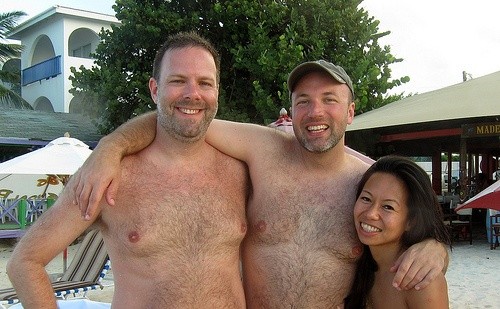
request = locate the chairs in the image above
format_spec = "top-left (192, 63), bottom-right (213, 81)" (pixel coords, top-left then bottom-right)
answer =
top-left (441, 191), bottom-right (500, 250)
top-left (0, 230), bottom-right (111, 309)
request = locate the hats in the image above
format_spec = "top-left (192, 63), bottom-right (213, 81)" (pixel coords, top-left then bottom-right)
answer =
top-left (287, 60), bottom-right (354, 102)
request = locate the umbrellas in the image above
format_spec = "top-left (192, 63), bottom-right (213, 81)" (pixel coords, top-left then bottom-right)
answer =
top-left (0, 132), bottom-right (93, 273)
top-left (455, 179), bottom-right (500, 212)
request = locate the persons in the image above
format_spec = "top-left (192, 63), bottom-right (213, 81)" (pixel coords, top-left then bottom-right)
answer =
top-left (7, 30), bottom-right (253, 309)
top-left (343, 155), bottom-right (449, 309)
top-left (70, 60), bottom-right (449, 309)
